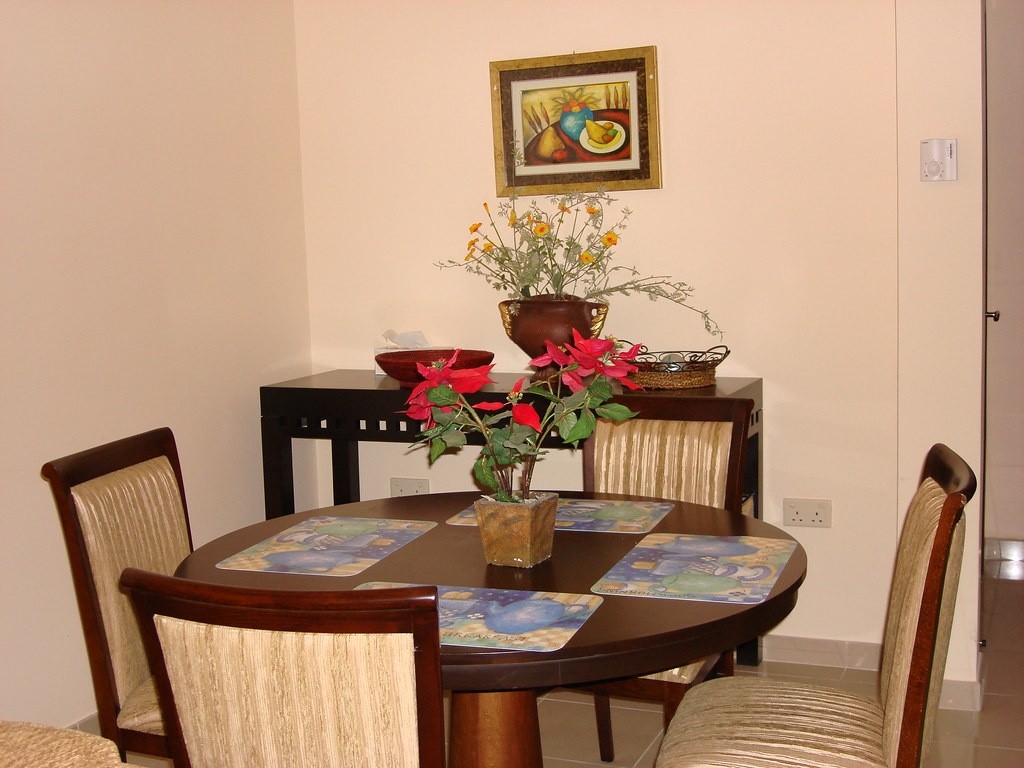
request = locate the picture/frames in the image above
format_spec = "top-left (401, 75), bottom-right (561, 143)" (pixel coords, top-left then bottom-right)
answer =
top-left (488, 46), bottom-right (662, 198)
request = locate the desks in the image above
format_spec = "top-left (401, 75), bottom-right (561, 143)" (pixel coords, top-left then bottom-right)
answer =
top-left (174, 491), bottom-right (808, 768)
top-left (261, 369), bottom-right (770, 526)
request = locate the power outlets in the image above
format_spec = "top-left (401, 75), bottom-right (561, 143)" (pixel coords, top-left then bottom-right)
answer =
top-left (783, 497), bottom-right (833, 528)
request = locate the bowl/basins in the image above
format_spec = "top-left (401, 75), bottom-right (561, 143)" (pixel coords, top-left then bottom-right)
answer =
top-left (375, 348), bottom-right (495, 389)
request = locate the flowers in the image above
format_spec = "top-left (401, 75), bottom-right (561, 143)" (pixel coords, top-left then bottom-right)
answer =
top-left (432, 130), bottom-right (734, 342)
top-left (392, 327), bottom-right (643, 500)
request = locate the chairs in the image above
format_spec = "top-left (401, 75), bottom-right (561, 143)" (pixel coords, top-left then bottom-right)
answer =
top-left (655, 443), bottom-right (977, 768)
top-left (119, 564), bottom-right (444, 768)
top-left (41, 427), bottom-right (222, 767)
top-left (581, 385), bottom-right (754, 761)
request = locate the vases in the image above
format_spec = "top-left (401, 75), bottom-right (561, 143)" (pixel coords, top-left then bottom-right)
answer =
top-left (498, 292), bottom-right (608, 382)
top-left (471, 489), bottom-right (560, 567)
